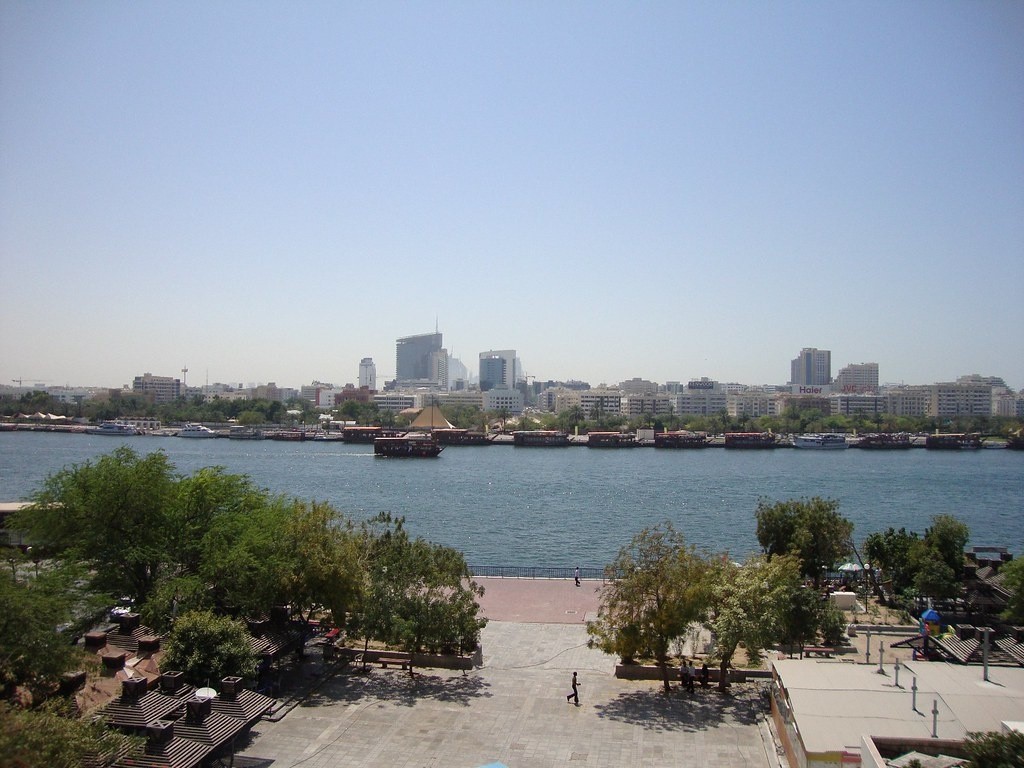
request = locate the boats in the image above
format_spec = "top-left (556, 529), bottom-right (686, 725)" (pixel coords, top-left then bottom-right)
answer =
top-left (430, 428), bottom-right (492, 446)
top-left (655, 431), bottom-right (711, 449)
top-left (724, 432), bottom-right (777, 449)
top-left (512, 430), bottom-right (571, 447)
top-left (151, 422), bottom-right (259, 440)
top-left (790, 432), bottom-right (849, 449)
top-left (586, 429), bottom-right (645, 448)
top-left (373, 437), bottom-right (442, 458)
top-left (84, 420), bottom-right (143, 437)
top-left (344, 425), bottom-right (397, 444)
top-left (926, 431), bottom-right (988, 448)
top-left (858, 429), bottom-right (914, 449)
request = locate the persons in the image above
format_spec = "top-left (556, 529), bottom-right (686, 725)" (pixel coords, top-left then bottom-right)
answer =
top-left (687, 661), bottom-right (695, 694)
top-left (567, 672), bottom-right (581, 707)
top-left (574, 567), bottom-right (581, 587)
top-left (681, 661), bottom-right (688, 688)
top-left (701, 664), bottom-right (710, 691)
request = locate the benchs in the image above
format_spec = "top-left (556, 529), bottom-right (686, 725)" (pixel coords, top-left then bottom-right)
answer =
top-left (676, 675), bottom-right (712, 683)
top-left (377, 658), bottom-right (410, 670)
top-left (308, 621), bottom-right (340, 643)
top-left (803, 647), bottom-right (834, 658)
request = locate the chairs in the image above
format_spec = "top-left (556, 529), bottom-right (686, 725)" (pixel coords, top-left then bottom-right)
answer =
top-left (353, 652), bottom-right (364, 666)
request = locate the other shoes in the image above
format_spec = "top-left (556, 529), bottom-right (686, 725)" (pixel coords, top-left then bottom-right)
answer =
top-left (575, 584), bottom-right (578, 585)
top-left (567, 696), bottom-right (569, 701)
top-left (579, 582), bottom-right (580, 584)
